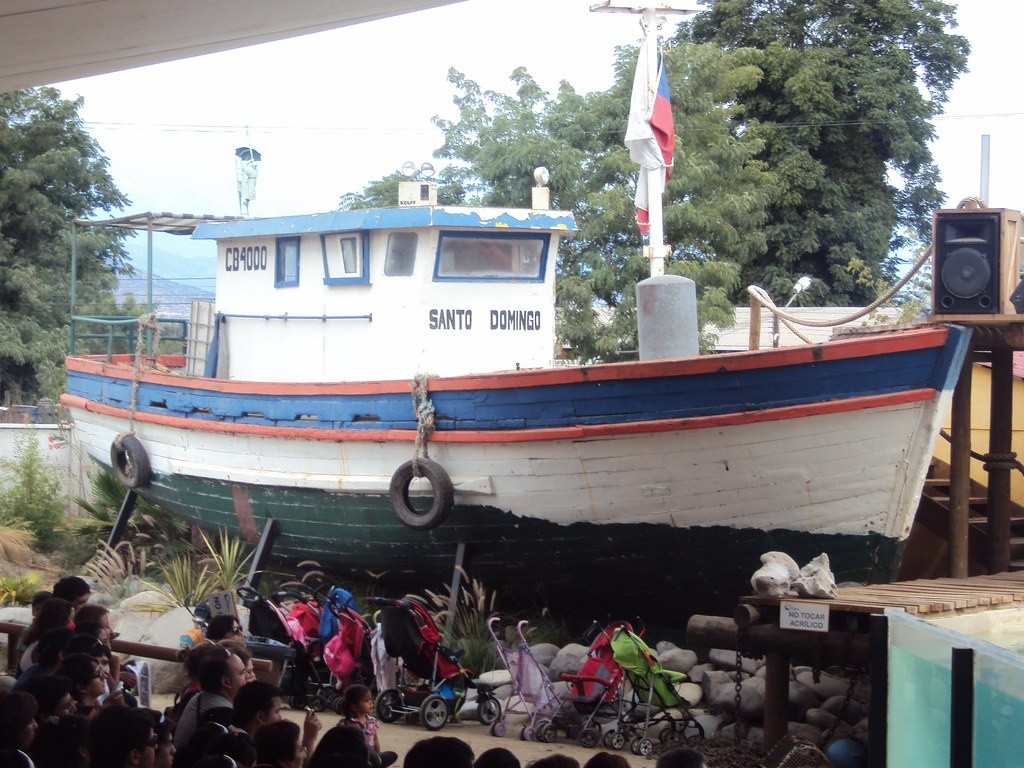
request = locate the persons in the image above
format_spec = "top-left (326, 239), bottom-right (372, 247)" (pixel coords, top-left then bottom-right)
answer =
top-left (336, 684), bottom-right (398, 768)
top-left (656, 747), bottom-right (707, 768)
top-left (474, 747), bottom-right (521, 768)
top-left (403, 736), bottom-right (475, 768)
top-left (307, 726), bottom-right (368, 768)
top-left (525, 753), bottom-right (581, 768)
top-left (583, 751), bottom-right (631, 768)
top-left (0, 576), bottom-right (322, 768)
top-left (253, 720), bottom-right (307, 768)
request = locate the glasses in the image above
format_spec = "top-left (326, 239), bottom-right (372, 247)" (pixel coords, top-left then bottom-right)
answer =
top-left (222, 755), bottom-right (237, 768)
top-left (223, 647), bottom-right (231, 657)
top-left (205, 638), bottom-right (217, 646)
top-left (93, 638), bottom-right (103, 648)
top-left (89, 665), bottom-right (103, 678)
top-left (206, 720), bottom-right (229, 737)
top-left (229, 626), bottom-right (242, 633)
top-left (145, 734), bottom-right (157, 747)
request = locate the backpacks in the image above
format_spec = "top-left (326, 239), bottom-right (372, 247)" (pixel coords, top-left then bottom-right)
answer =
top-left (287, 617), bottom-right (310, 649)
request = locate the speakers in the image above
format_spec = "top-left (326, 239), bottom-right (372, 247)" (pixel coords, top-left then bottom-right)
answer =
top-left (930, 208), bottom-right (1021, 323)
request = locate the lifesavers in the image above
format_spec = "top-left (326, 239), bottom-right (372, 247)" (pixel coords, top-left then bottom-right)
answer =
top-left (109, 433), bottom-right (152, 490)
top-left (389, 457), bottom-right (456, 533)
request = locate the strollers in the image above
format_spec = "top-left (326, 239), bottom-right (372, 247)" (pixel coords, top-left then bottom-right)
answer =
top-left (176, 562), bottom-right (707, 758)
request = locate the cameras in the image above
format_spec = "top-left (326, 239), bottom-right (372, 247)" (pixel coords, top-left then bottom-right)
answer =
top-left (306, 708), bottom-right (314, 717)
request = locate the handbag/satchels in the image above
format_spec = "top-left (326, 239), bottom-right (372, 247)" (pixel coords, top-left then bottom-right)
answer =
top-left (323, 636), bottom-right (356, 679)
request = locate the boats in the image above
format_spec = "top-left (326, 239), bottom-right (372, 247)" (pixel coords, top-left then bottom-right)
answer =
top-left (59, 205), bottom-right (975, 647)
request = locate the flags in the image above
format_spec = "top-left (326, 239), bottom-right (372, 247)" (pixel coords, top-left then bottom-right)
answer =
top-left (622, 37), bottom-right (675, 237)
